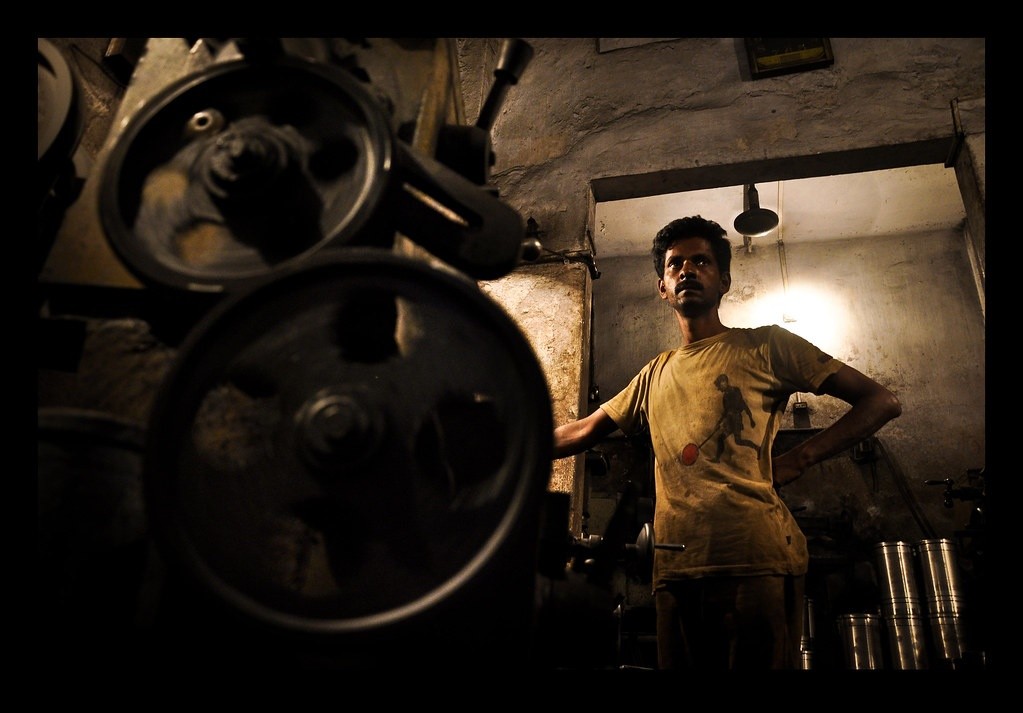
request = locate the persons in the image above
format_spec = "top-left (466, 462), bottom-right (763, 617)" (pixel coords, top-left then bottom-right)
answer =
top-left (554, 216), bottom-right (904, 713)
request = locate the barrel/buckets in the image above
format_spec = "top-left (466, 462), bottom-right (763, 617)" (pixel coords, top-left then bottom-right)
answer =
top-left (839, 537), bottom-right (968, 671)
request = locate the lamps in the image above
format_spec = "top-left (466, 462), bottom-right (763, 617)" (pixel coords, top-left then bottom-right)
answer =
top-left (733, 182), bottom-right (780, 238)
